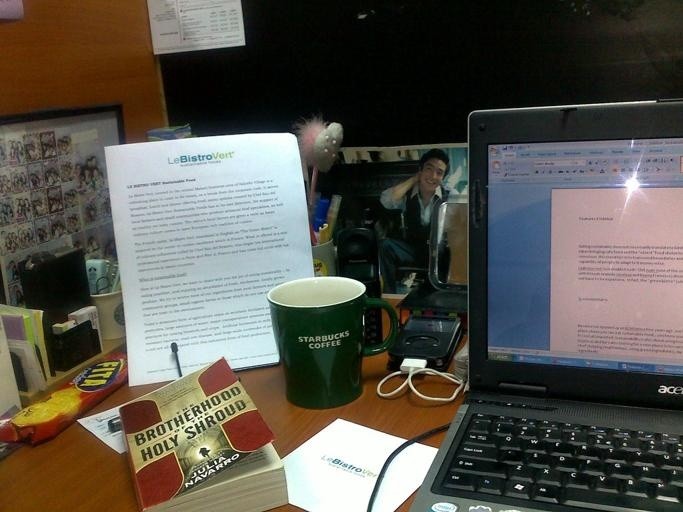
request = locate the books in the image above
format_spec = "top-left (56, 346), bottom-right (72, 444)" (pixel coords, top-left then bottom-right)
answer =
top-left (119, 357), bottom-right (290, 512)
top-left (0, 304), bottom-right (54, 414)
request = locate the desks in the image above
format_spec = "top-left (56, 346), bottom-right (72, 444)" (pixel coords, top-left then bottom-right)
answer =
top-left (0, 296), bottom-right (472, 512)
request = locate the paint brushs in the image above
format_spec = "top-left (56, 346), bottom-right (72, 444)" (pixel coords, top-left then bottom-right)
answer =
top-left (328, 195), bottom-right (342, 238)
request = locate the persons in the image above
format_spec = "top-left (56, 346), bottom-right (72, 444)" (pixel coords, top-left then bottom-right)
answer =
top-left (374, 148), bottom-right (463, 296)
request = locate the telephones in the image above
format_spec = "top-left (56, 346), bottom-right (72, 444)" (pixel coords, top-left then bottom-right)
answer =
top-left (338, 216), bottom-right (383, 347)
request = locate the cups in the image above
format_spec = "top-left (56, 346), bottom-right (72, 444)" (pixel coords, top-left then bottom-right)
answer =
top-left (267, 277), bottom-right (398, 409)
top-left (90, 290), bottom-right (125, 340)
top-left (311, 238), bottom-right (337, 277)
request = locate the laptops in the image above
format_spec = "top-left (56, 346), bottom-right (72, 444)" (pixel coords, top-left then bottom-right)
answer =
top-left (411, 97), bottom-right (683, 511)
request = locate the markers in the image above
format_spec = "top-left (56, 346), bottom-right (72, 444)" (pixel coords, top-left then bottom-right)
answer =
top-left (313, 200), bottom-right (329, 242)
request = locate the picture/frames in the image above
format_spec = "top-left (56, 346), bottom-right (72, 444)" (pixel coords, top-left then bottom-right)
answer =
top-left (1, 103), bottom-right (128, 304)
